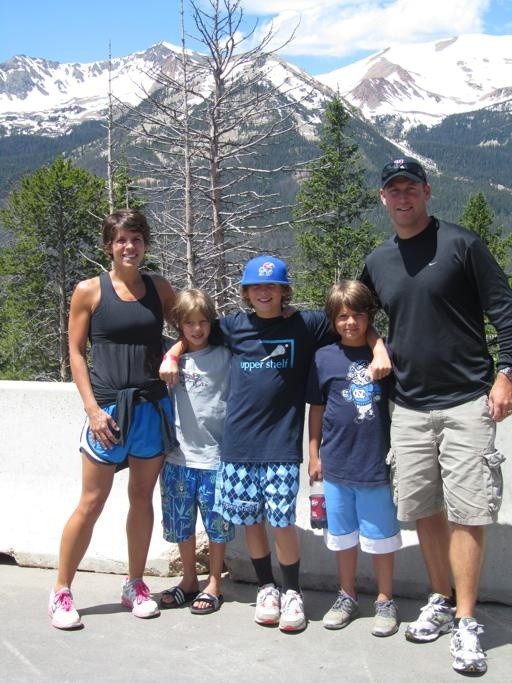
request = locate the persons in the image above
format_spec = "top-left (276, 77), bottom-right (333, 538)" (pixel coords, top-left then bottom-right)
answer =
top-left (155, 285), bottom-right (236, 619)
top-left (159, 253), bottom-right (393, 632)
top-left (303, 282), bottom-right (400, 634)
top-left (358, 159), bottom-right (512, 672)
top-left (50, 208), bottom-right (193, 633)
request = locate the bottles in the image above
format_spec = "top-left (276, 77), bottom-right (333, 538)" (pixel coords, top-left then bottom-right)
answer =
top-left (309, 480), bottom-right (328, 528)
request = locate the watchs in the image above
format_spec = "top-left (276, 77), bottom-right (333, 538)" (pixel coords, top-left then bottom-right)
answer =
top-left (496, 363), bottom-right (512, 381)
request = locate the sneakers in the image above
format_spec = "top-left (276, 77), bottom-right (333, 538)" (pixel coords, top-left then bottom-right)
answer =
top-left (48, 587), bottom-right (81, 630)
top-left (322, 590), bottom-right (361, 629)
top-left (254, 583), bottom-right (280, 626)
top-left (370, 599), bottom-right (400, 637)
top-left (448, 616), bottom-right (488, 673)
top-left (279, 589), bottom-right (306, 631)
top-left (121, 577), bottom-right (160, 619)
top-left (405, 593), bottom-right (456, 643)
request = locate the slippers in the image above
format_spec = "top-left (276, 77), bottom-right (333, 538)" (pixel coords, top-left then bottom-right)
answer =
top-left (160, 586), bottom-right (201, 609)
top-left (190, 593), bottom-right (225, 615)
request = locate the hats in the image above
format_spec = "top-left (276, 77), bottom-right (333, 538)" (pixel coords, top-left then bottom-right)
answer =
top-left (380, 157), bottom-right (427, 190)
top-left (241, 255), bottom-right (291, 286)
